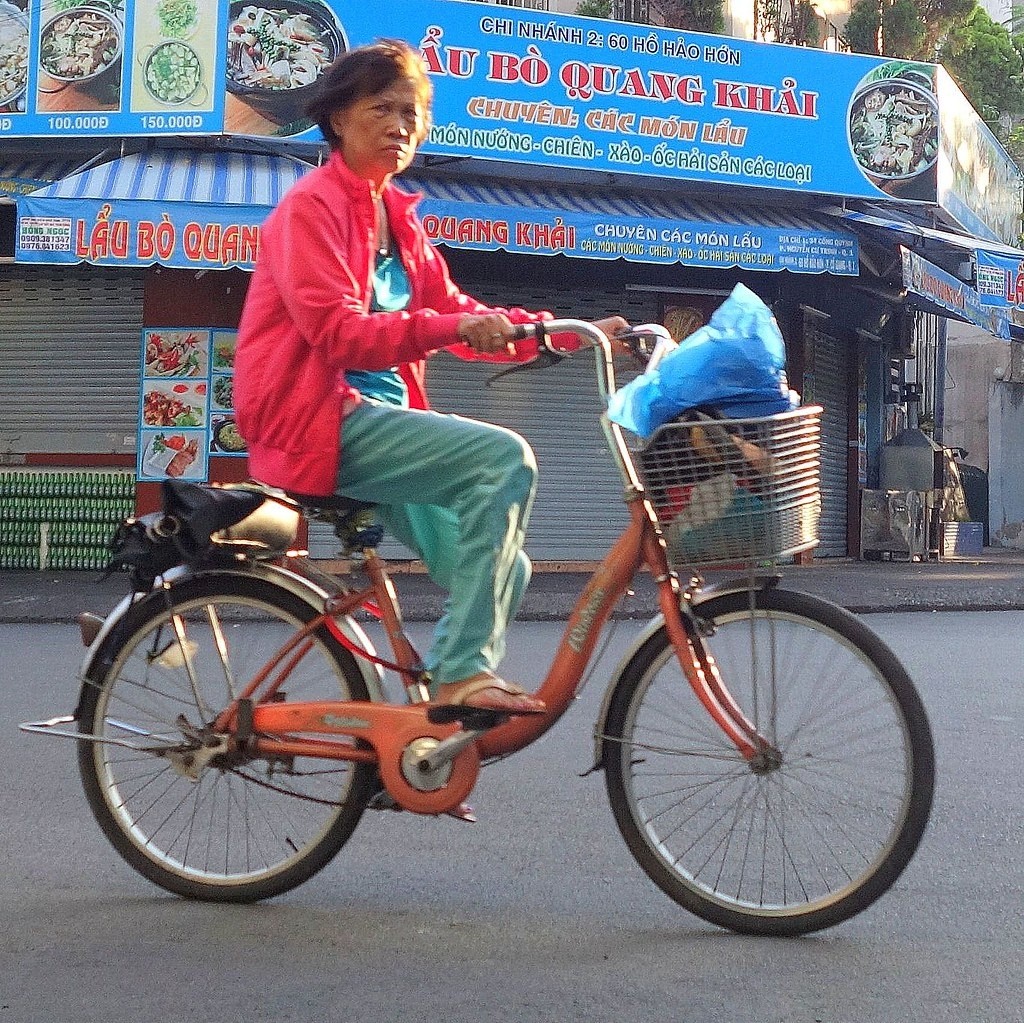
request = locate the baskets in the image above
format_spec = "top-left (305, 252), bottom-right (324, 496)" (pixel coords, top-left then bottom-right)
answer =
top-left (628, 401), bottom-right (825, 570)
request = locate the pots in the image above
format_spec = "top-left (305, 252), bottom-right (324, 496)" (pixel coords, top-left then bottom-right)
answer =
top-left (138, 40), bottom-right (208, 107)
top-left (227, 0), bottom-right (349, 110)
top-left (0, 0), bottom-right (29, 106)
top-left (211, 419), bottom-right (247, 452)
top-left (38, 0), bottom-right (123, 93)
top-left (851, 71), bottom-right (938, 180)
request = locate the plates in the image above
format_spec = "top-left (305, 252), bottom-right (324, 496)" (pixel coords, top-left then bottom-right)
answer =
top-left (143, 434), bottom-right (162, 476)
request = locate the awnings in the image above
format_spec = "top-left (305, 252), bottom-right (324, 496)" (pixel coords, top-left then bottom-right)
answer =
top-left (0, 148), bottom-right (1024, 343)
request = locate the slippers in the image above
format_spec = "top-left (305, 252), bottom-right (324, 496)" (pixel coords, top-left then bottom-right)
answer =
top-left (425, 675), bottom-right (547, 723)
top-left (447, 802), bottom-right (479, 822)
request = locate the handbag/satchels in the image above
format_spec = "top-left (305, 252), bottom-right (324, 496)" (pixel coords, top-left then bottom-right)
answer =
top-left (609, 282), bottom-right (806, 438)
top-left (99, 478), bottom-right (306, 602)
top-left (677, 407), bottom-right (802, 509)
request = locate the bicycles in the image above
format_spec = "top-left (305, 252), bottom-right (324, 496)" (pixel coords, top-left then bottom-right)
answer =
top-left (18, 323), bottom-right (936, 938)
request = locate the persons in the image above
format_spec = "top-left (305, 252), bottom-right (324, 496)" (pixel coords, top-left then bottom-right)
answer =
top-left (234, 41), bottom-right (629, 822)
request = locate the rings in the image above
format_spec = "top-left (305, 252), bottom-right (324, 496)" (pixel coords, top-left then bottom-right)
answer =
top-left (490, 333), bottom-right (501, 339)
